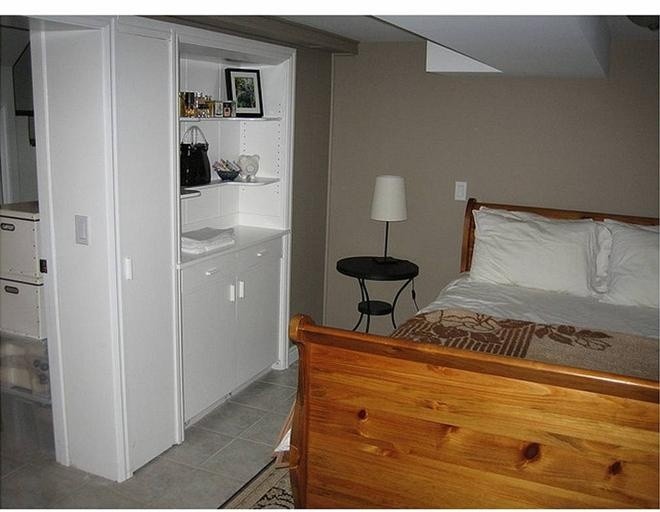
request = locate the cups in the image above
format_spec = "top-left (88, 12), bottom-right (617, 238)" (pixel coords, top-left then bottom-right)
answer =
top-left (214, 101), bottom-right (224, 119)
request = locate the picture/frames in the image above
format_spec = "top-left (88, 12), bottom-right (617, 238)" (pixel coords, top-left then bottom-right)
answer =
top-left (224, 67), bottom-right (264, 118)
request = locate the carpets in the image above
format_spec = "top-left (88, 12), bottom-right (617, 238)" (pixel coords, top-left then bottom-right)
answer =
top-left (216, 457), bottom-right (295, 509)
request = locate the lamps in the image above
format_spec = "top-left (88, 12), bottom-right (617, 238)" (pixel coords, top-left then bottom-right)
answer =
top-left (369, 176), bottom-right (406, 265)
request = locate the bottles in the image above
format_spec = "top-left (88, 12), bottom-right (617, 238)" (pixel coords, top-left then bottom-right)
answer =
top-left (178, 90), bottom-right (215, 118)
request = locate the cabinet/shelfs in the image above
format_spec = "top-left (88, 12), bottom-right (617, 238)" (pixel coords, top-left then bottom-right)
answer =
top-left (176, 24), bottom-right (298, 428)
top-left (26, 16), bottom-right (181, 482)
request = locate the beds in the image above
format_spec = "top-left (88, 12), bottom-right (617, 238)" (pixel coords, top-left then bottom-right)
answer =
top-left (269, 196), bottom-right (660, 509)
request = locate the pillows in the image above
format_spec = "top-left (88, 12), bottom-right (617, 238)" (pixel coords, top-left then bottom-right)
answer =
top-left (471, 206), bottom-right (660, 310)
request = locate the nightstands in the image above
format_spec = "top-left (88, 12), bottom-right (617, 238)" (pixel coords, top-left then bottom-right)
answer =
top-left (336, 256), bottom-right (419, 334)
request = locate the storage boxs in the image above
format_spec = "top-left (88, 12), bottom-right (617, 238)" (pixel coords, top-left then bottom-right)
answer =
top-left (0, 200), bottom-right (51, 407)
